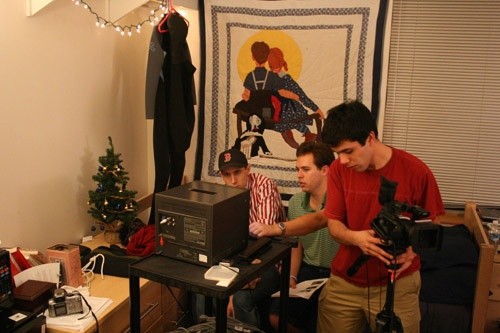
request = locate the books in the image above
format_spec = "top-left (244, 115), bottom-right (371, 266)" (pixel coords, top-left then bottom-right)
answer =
top-left (38, 296), bottom-right (112, 332)
top-left (6, 246), bottom-right (50, 287)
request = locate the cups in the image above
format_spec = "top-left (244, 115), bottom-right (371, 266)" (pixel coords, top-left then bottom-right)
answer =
top-left (488, 223), bottom-right (500, 255)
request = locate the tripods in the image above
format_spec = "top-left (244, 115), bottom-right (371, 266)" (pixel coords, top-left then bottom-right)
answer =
top-left (346, 245), bottom-right (404, 333)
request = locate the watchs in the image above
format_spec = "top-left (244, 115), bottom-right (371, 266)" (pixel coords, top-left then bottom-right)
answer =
top-left (279, 223), bottom-right (286, 236)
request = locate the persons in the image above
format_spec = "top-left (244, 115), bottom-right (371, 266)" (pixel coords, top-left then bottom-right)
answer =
top-left (249, 142), bottom-right (336, 333)
top-left (317, 102), bottom-right (446, 333)
top-left (218, 149), bottom-right (286, 321)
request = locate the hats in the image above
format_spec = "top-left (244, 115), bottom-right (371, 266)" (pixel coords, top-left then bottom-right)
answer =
top-left (218, 148), bottom-right (248, 170)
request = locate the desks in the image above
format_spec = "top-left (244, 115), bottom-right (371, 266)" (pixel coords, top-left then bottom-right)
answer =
top-left (129, 240), bottom-right (291, 333)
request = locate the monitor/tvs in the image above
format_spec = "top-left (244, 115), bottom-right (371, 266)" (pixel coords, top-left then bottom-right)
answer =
top-left (153, 179), bottom-right (251, 269)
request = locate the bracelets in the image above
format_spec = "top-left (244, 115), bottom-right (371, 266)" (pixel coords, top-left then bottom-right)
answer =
top-left (290, 276), bottom-right (298, 282)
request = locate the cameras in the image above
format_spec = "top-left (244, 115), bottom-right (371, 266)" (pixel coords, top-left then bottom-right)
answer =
top-left (48, 289), bottom-right (83, 318)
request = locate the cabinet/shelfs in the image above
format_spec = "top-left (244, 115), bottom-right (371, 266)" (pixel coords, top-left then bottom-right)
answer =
top-left (44, 231), bottom-right (188, 333)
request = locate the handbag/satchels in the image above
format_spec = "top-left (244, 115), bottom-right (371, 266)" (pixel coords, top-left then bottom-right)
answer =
top-left (413, 223), bottom-right (479, 304)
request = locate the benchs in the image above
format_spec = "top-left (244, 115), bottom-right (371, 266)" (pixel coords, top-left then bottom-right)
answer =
top-left (130, 175), bottom-right (495, 333)
